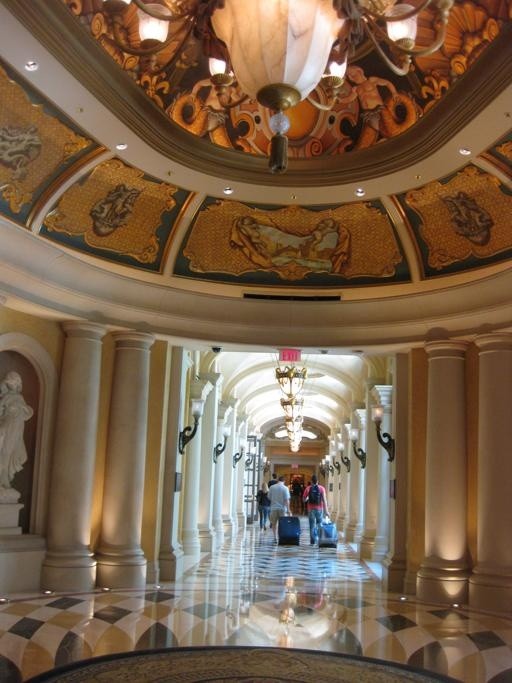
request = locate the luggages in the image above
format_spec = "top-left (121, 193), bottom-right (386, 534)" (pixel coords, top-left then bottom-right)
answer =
top-left (278, 511), bottom-right (300, 545)
top-left (319, 515), bottom-right (338, 548)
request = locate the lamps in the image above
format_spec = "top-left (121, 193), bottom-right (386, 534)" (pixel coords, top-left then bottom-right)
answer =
top-left (369, 403), bottom-right (394, 461)
top-left (177, 397), bottom-right (204, 454)
top-left (211, 425), bottom-right (268, 471)
top-left (94, 0), bottom-right (456, 175)
top-left (274, 345), bottom-right (311, 451)
top-left (320, 427), bottom-right (366, 476)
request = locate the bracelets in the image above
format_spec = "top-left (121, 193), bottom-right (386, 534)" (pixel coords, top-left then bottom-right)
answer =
top-left (326, 512), bottom-right (330, 516)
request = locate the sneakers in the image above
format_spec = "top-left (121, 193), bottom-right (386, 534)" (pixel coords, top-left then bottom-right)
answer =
top-left (274, 539), bottom-right (277, 542)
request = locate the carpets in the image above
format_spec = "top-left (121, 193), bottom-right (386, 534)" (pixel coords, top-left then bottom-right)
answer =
top-left (15, 644), bottom-right (466, 681)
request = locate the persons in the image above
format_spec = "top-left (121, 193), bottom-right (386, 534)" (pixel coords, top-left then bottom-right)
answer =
top-left (269, 474), bottom-right (278, 528)
top-left (302, 475), bottom-right (330, 545)
top-left (288, 476), bottom-right (306, 514)
top-left (256, 483), bottom-right (269, 530)
top-left (268, 476), bottom-right (292, 544)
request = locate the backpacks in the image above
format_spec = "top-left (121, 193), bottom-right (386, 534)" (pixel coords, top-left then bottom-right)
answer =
top-left (309, 483), bottom-right (321, 504)
